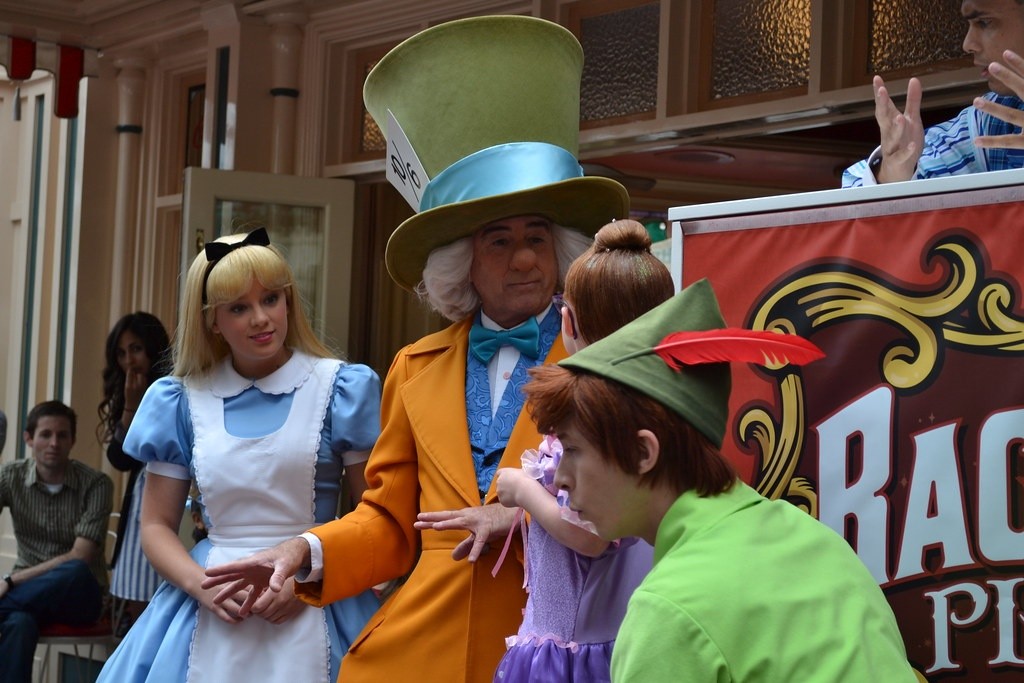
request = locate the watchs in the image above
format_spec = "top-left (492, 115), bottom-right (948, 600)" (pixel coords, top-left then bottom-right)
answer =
top-left (2, 573), bottom-right (14, 589)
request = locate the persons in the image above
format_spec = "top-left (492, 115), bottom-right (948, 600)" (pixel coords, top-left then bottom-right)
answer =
top-left (496, 218), bottom-right (674, 683)
top-left (96, 310), bottom-right (208, 640)
top-left (0, 399), bottom-right (114, 683)
top-left (842, 0), bottom-right (1024, 189)
top-left (201, 14), bottom-right (631, 683)
top-left (522, 277), bottom-right (919, 683)
top-left (95, 227), bottom-right (381, 683)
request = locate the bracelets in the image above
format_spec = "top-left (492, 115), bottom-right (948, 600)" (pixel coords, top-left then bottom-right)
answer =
top-left (124, 409), bottom-right (135, 411)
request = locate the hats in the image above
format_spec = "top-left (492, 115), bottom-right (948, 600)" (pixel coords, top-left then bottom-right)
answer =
top-left (363, 15), bottom-right (630, 298)
top-left (557, 276), bottom-right (826, 449)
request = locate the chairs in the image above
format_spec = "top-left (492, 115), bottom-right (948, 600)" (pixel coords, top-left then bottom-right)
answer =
top-left (37, 513), bottom-right (121, 683)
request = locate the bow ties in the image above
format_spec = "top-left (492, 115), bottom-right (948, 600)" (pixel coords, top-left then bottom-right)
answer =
top-left (468, 314), bottom-right (541, 365)
